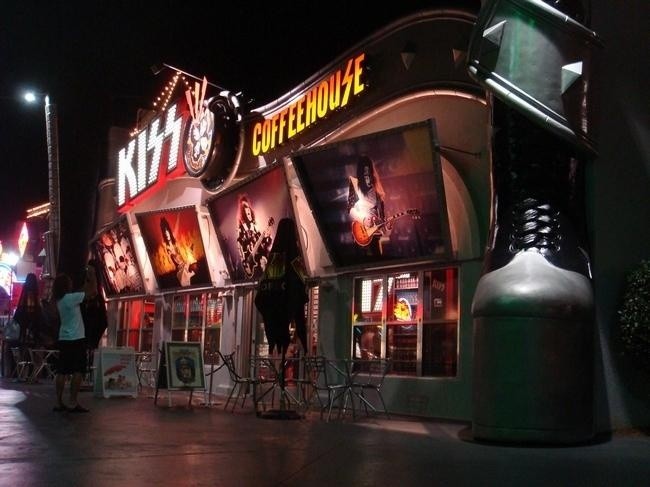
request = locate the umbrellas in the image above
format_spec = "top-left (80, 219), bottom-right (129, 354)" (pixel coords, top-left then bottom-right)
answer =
top-left (254, 217), bottom-right (308, 411)
top-left (12, 272), bottom-right (45, 358)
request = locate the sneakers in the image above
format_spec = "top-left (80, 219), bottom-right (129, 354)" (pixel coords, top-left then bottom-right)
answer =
top-left (50, 401), bottom-right (91, 416)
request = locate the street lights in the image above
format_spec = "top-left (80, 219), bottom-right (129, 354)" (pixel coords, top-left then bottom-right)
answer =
top-left (7, 252), bottom-right (19, 324)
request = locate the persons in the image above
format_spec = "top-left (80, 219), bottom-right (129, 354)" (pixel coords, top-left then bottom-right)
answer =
top-left (160, 216), bottom-right (198, 286)
top-left (236, 192), bottom-right (275, 280)
top-left (52, 258), bottom-right (97, 413)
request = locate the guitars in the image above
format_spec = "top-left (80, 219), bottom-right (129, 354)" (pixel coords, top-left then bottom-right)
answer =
top-left (240, 216), bottom-right (275, 277)
top-left (352, 208), bottom-right (422, 247)
top-left (177, 241), bottom-right (195, 286)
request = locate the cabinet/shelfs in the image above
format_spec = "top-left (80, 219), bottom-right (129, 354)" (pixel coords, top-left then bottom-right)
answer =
top-left (392, 273), bottom-right (444, 377)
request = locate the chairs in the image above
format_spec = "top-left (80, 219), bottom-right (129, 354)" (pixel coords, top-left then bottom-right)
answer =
top-left (222, 354), bottom-right (393, 423)
top-left (9, 343), bottom-right (162, 394)
